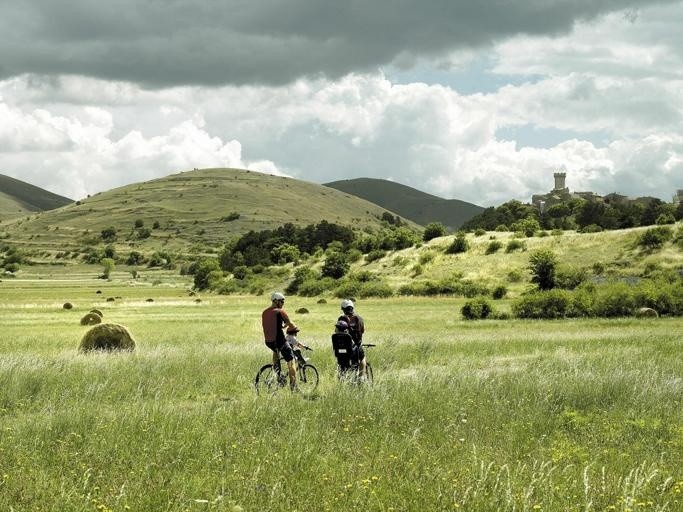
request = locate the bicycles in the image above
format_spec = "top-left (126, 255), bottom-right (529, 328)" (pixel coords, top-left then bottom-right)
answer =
top-left (252, 350), bottom-right (319, 397)
top-left (331, 331), bottom-right (376, 389)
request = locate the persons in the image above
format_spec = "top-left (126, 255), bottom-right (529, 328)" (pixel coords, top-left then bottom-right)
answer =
top-left (334, 321), bottom-right (360, 366)
top-left (262, 291), bottom-right (303, 394)
top-left (337, 315), bottom-right (360, 348)
top-left (284, 325), bottom-right (307, 376)
top-left (334, 298), bottom-right (367, 386)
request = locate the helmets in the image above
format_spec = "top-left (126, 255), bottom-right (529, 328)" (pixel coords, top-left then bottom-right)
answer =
top-left (287, 326), bottom-right (299, 334)
top-left (338, 315), bottom-right (350, 323)
top-left (270, 292), bottom-right (285, 302)
top-left (334, 320), bottom-right (349, 329)
top-left (340, 299), bottom-right (354, 310)
